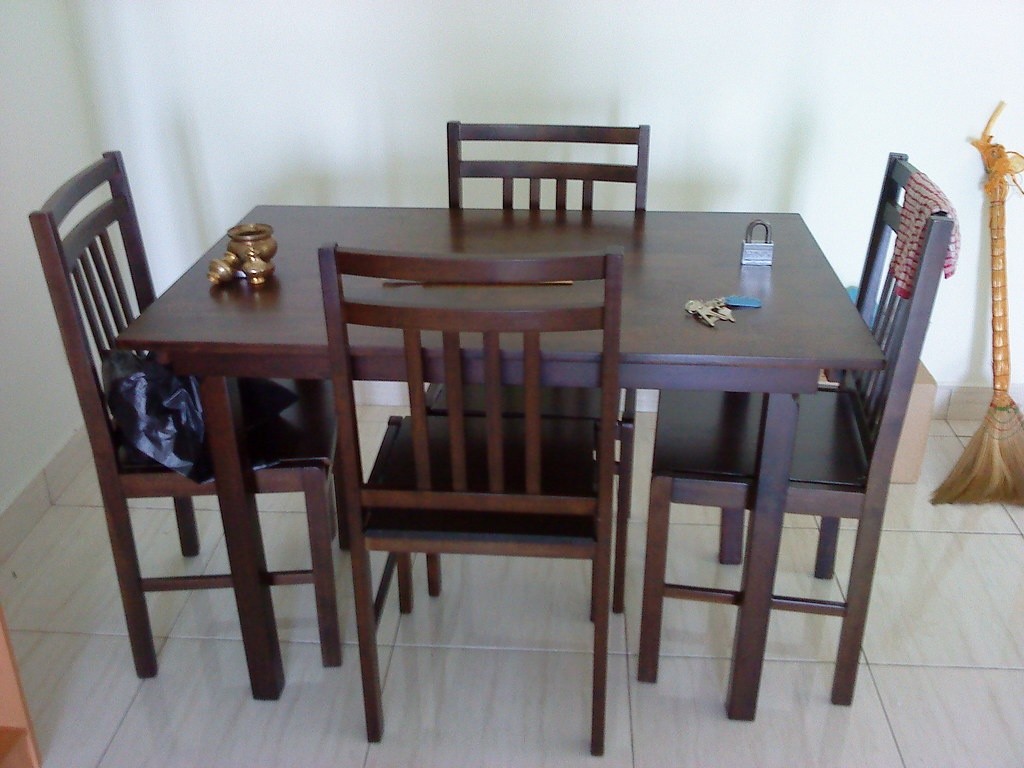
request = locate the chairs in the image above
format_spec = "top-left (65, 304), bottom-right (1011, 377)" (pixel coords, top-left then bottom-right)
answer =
top-left (318, 243), bottom-right (621, 758)
top-left (637, 153), bottom-right (954, 706)
top-left (27, 150), bottom-right (345, 681)
top-left (424, 122), bottom-right (651, 614)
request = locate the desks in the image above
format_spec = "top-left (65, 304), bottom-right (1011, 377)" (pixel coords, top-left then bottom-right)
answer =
top-left (114, 205), bottom-right (883, 722)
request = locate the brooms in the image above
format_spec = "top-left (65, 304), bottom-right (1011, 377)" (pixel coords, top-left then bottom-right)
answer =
top-left (926, 100), bottom-right (1024, 510)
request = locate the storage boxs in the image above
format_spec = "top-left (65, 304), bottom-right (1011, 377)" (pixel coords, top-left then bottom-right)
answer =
top-left (820, 360), bottom-right (936, 485)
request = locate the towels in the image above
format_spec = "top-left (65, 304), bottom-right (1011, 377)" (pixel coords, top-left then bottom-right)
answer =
top-left (887, 172), bottom-right (961, 300)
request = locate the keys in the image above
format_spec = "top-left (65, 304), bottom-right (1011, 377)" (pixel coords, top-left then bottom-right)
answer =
top-left (684, 296), bottom-right (735, 327)
